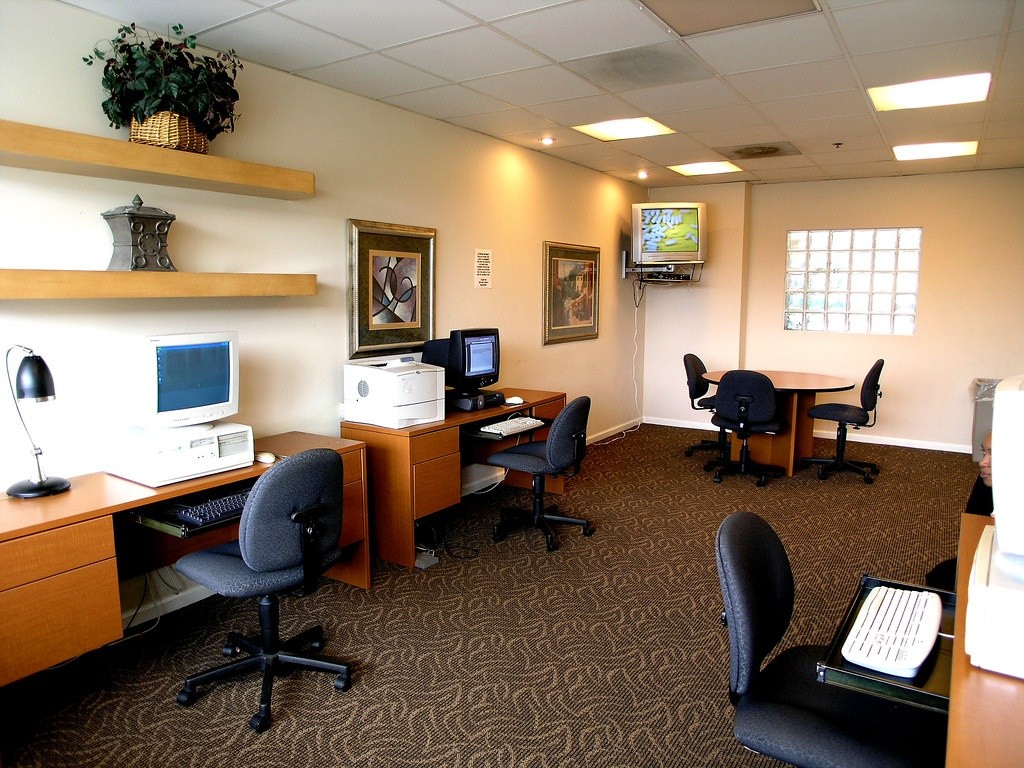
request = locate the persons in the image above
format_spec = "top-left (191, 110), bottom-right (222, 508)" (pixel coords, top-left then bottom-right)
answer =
top-left (925, 432), bottom-right (994, 592)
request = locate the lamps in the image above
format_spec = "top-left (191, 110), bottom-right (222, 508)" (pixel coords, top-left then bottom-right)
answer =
top-left (6, 345), bottom-right (71, 501)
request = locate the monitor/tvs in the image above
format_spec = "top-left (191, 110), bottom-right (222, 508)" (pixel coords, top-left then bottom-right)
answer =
top-left (422, 329), bottom-right (500, 397)
top-left (631, 202), bottom-right (707, 265)
top-left (141, 331), bottom-right (239, 434)
top-left (991, 374), bottom-right (1024, 582)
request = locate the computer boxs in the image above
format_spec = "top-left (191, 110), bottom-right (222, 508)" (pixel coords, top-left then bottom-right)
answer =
top-left (101, 420), bottom-right (255, 488)
top-left (964, 525), bottom-right (1024, 678)
top-left (445, 390), bottom-right (505, 413)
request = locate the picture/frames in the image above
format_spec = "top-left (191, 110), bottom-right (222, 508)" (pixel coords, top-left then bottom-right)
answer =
top-left (345, 218), bottom-right (437, 361)
top-left (541, 239), bottom-right (601, 347)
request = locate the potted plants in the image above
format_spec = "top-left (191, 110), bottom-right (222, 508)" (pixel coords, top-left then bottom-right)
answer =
top-left (81, 17), bottom-right (245, 157)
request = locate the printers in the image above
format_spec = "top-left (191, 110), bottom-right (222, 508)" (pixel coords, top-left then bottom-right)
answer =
top-left (343, 355), bottom-right (445, 429)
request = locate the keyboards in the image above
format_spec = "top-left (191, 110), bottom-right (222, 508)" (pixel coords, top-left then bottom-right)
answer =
top-left (480, 417), bottom-right (545, 437)
top-left (841, 586), bottom-right (942, 678)
top-left (176, 492), bottom-right (251, 526)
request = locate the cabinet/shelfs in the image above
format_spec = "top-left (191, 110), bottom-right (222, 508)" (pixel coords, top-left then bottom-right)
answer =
top-left (0, 119), bottom-right (318, 301)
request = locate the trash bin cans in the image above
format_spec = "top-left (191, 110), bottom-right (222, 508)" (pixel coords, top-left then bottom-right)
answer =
top-left (971, 377), bottom-right (1002, 463)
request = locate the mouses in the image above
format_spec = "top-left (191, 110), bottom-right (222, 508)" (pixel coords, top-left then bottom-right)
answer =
top-left (506, 397), bottom-right (523, 404)
top-left (254, 452), bottom-right (275, 463)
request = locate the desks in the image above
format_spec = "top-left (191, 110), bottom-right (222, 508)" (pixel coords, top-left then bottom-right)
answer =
top-left (0, 431), bottom-right (371, 688)
top-left (700, 369), bottom-right (855, 480)
top-left (943, 512), bottom-right (1024, 768)
top-left (339, 386), bottom-right (566, 570)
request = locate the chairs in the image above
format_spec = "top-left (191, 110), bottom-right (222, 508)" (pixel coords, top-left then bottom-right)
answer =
top-left (711, 369), bottom-right (787, 488)
top-left (713, 511), bottom-right (945, 768)
top-left (172, 448), bottom-right (356, 734)
top-left (487, 396), bottom-right (595, 552)
top-left (799, 359), bottom-right (887, 485)
top-left (683, 354), bottom-right (731, 458)
